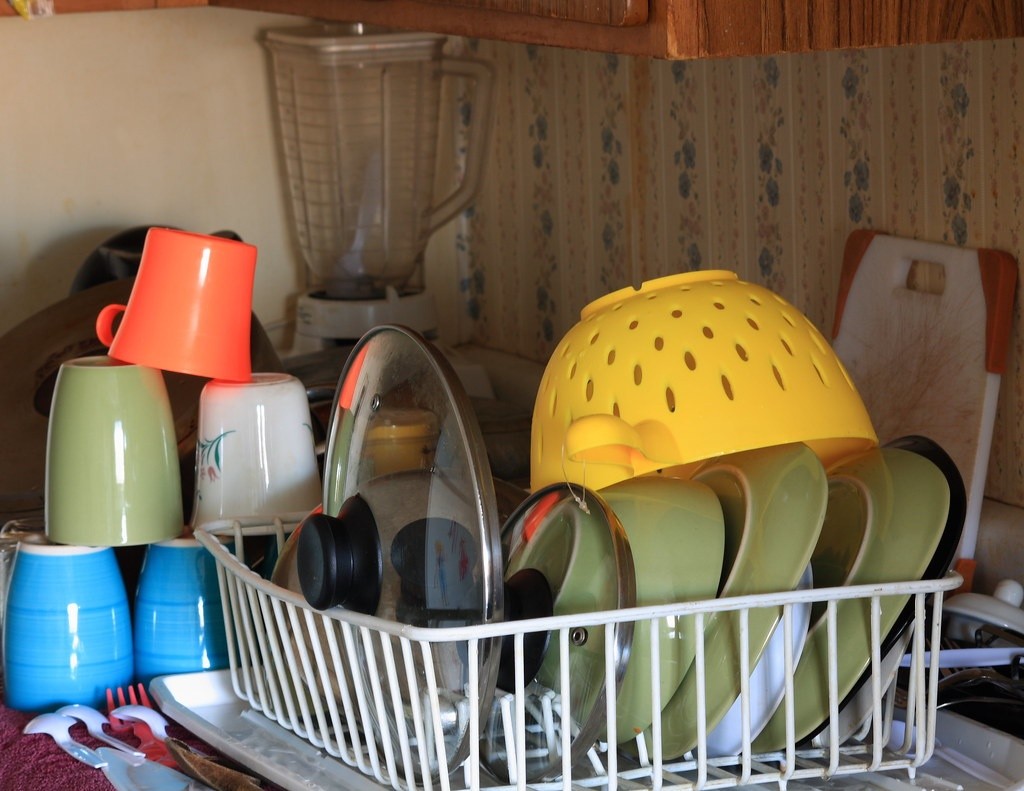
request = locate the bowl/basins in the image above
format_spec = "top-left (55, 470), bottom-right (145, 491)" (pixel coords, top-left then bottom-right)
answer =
top-left (504, 473), bottom-right (729, 749)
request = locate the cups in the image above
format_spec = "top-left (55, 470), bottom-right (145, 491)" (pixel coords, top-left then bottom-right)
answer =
top-left (43, 353), bottom-right (189, 555)
top-left (192, 371), bottom-right (325, 537)
top-left (95, 225), bottom-right (258, 384)
top-left (0, 539), bottom-right (135, 715)
top-left (127, 537), bottom-right (239, 681)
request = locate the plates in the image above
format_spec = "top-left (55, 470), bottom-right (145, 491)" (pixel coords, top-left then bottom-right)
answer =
top-left (816, 602), bottom-right (927, 749)
top-left (696, 560), bottom-right (817, 762)
top-left (797, 433), bottom-right (971, 751)
top-left (743, 444), bottom-right (952, 757)
top-left (632, 431), bottom-right (832, 762)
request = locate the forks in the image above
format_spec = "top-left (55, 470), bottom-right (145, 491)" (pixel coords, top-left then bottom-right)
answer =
top-left (104, 682), bottom-right (184, 771)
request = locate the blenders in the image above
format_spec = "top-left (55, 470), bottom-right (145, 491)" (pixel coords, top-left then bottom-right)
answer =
top-left (262, 18), bottom-right (498, 398)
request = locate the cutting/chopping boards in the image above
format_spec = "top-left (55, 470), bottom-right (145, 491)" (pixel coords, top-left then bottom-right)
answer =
top-left (827, 228), bottom-right (1019, 604)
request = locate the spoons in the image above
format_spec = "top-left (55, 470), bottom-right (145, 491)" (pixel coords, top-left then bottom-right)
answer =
top-left (56, 703), bottom-right (144, 760)
top-left (110, 704), bottom-right (171, 740)
top-left (24, 710), bottom-right (108, 769)
